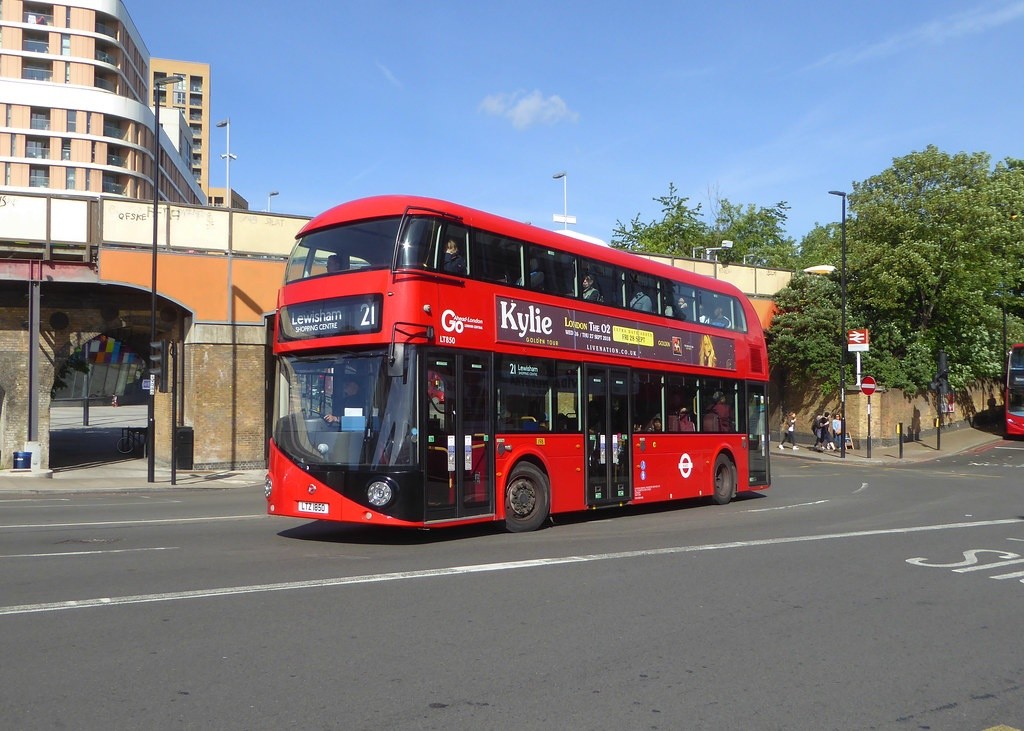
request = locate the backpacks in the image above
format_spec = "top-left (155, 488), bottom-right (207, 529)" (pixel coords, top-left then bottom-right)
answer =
top-left (816, 419), bottom-right (821, 429)
top-left (811, 425), bottom-right (817, 436)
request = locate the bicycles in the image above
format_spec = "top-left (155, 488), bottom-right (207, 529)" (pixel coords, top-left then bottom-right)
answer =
top-left (116, 430), bottom-right (144, 454)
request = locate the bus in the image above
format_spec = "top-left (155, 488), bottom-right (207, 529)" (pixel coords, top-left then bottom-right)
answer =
top-left (1006, 344), bottom-right (1024, 435)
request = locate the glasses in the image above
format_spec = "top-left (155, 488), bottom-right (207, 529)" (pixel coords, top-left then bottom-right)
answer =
top-left (583, 277), bottom-right (589, 282)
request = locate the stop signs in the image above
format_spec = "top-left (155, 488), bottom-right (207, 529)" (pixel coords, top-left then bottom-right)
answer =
top-left (861, 376), bottom-right (876, 395)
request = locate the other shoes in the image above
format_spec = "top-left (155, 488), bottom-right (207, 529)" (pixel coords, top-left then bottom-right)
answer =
top-left (792, 446), bottom-right (799, 450)
top-left (814, 445), bottom-right (841, 452)
top-left (779, 444), bottom-right (784, 449)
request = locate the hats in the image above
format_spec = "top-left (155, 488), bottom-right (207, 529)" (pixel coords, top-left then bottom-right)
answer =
top-left (712, 391), bottom-right (726, 401)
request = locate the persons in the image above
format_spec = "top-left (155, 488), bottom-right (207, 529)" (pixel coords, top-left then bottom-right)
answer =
top-left (630, 282), bottom-right (652, 312)
top-left (648, 413), bottom-right (662, 432)
top-left (324, 381), bottom-right (364, 423)
top-left (779, 412), bottom-right (799, 450)
top-left (582, 276), bottom-right (599, 301)
top-left (451, 410), bottom-right (567, 433)
top-left (709, 305), bottom-right (729, 329)
top-left (673, 297), bottom-right (690, 319)
top-left (987, 394), bottom-right (998, 428)
top-left (441, 240), bottom-right (466, 274)
top-left (814, 412), bottom-right (841, 450)
top-left (516, 258), bottom-right (544, 290)
top-left (698, 335), bottom-right (718, 367)
top-left (699, 305), bottom-right (709, 324)
top-left (112, 394), bottom-right (118, 407)
top-left (677, 408), bottom-right (695, 432)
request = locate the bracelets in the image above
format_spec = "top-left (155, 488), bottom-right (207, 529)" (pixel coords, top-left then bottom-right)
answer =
top-left (336, 416), bottom-right (338, 419)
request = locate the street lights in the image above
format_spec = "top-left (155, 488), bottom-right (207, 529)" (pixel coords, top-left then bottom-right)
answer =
top-left (216, 116), bottom-right (232, 207)
top-left (267, 190), bottom-right (279, 211)
top-left (262, 193), bottom-right (767, 534)
top-left (150, 73), bottom-right (186, 484)
top-left (552, 172), bottom-right (567, 234)
top-left (828, 189), bottom-right (850, 458)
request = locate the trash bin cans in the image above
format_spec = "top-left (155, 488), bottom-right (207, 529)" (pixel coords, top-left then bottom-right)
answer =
top-left (12, 452), bottom-right (31, 468)
